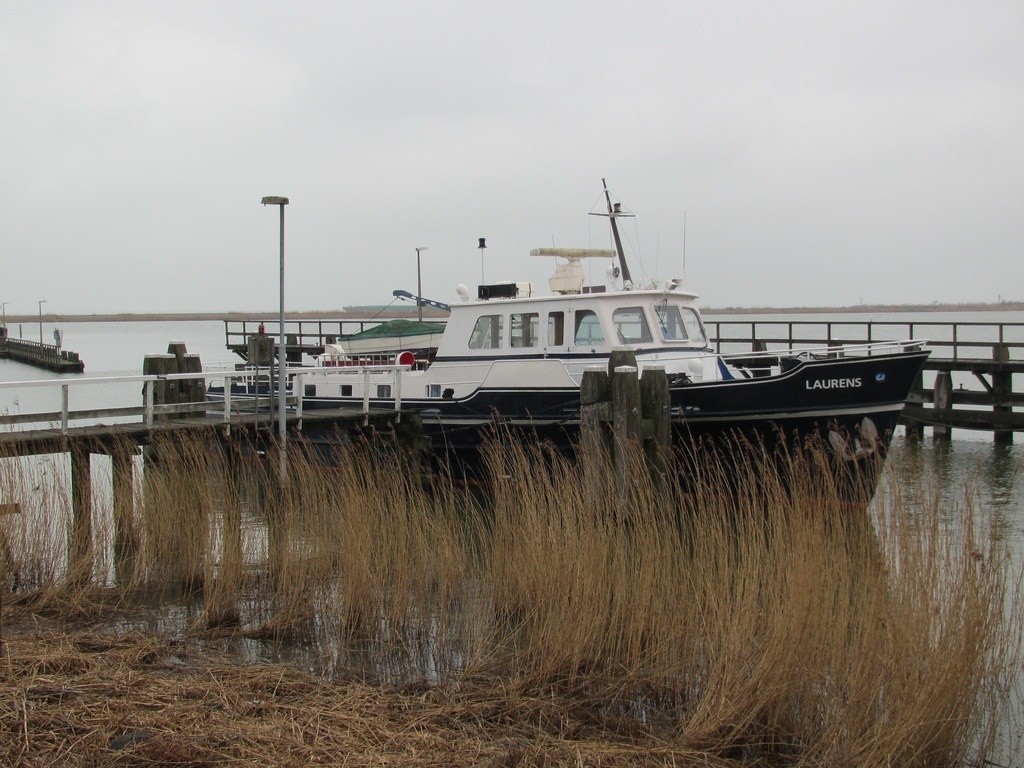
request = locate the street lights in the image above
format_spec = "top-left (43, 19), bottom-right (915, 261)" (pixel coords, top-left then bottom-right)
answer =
top-left (261, 196), bottom-right (290, 494)
top-left (39, 300), bottom-right (47, 357)
top-left (416, 248), bottom-right (427, 321)
top-left (3, 302), bottom-right (9, 340)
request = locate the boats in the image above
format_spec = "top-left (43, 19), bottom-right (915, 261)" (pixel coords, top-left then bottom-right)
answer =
top-left (206, 179), bottom-right (934, 515)
